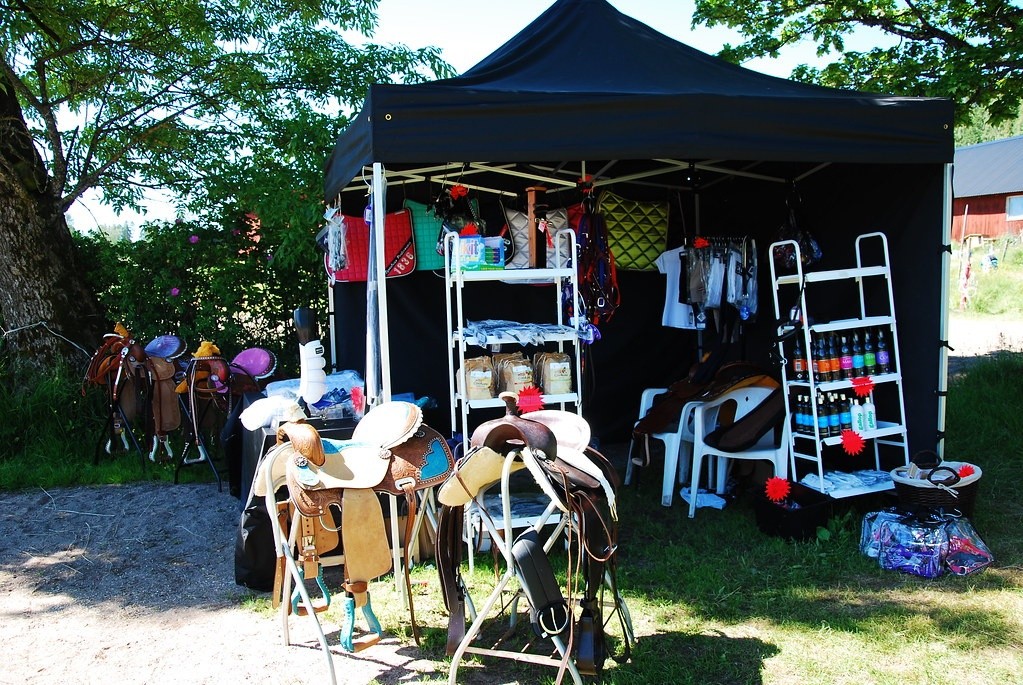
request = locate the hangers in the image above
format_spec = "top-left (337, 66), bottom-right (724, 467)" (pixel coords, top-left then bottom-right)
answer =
top-left (683, 233), bottom-right (746, 251)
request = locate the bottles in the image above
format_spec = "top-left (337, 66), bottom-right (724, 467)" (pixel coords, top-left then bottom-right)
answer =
top-left (795, 330), bottom-right (890, 440)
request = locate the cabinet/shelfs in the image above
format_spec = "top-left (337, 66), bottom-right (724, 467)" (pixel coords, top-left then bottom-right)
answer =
top-left (769, 232), bottom-right (910, 500)
top-left (444, 228), bottom-right (585, 573)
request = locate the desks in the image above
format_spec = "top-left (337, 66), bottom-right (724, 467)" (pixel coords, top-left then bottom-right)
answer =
top-left (219, 391), bottom-right (360, 514)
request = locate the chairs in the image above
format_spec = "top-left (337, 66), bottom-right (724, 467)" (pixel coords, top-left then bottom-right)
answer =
top-left (623, 387), bottom-right (722, 511)
top-left (688, 387), bottom-right (793, 518)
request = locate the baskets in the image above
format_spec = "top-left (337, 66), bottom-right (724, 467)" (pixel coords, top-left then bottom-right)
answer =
top-left (891, 450), bottom-right (982, 520)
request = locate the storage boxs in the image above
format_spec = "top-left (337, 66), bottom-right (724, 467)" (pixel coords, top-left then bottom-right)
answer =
top-left (750, 480), bottom-right (831, 544)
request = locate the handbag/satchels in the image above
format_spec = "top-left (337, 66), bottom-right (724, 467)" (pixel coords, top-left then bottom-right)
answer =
top-left (505, 207), bottom-right (569, 268)
top-left (403, 198), bottom-right (480, 271)
top-left (438, 196), bottom-right (515, 264)
top-left (594, 191), bottom-right (671, 272)
top-left (861, 510), bottom-right (994, 576)
top-left (324, 209), bottom-right (416, 282)
